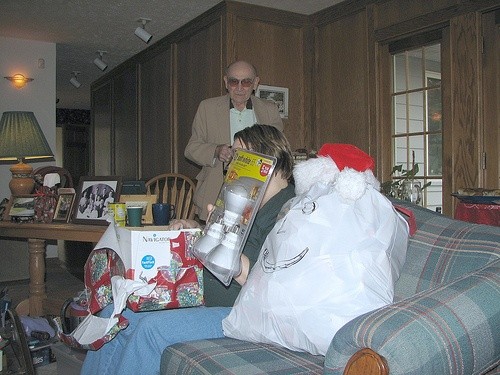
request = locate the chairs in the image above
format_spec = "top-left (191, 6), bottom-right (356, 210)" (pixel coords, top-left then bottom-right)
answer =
top-left (144, 174), bottom-right (196, 221)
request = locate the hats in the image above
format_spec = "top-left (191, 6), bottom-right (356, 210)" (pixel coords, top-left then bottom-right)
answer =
top-left (291, 142), bottom-right (381, 200)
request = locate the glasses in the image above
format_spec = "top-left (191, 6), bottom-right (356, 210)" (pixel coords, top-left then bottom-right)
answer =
top-left (227, 76), bottom-right (256, 87)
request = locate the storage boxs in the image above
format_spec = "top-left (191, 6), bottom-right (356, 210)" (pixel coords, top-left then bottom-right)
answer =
top-left (115, 225), bottom-right (204, 312)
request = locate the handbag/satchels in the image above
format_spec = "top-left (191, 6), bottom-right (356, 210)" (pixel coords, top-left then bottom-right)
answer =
top-left (221, 181), bottom-right (416, 356)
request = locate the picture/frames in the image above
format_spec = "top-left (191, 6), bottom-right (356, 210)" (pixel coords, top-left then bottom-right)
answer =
top-left (73, 175), bottom-right (124, 225)
top-left (254, 84), bottom-right (289, 120)
top-left (52, 194), bottom-right (76, 223)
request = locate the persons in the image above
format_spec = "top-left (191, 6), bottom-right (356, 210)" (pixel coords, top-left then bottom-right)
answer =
top-left (80, 124), bottom-right (295, 375)
top-left (184, 61), bottom-right (284, 224)
top-left (77, 187), bottom-right (115, 218)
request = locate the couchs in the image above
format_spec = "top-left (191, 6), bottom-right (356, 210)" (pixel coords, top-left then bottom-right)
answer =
top-left (161, 200), bottom-right (500, 375)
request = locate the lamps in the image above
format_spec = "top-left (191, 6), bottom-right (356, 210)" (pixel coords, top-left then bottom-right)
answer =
top-left (0, 111), bottom-right (54, 197)
top-left (94, 51), bottom-right (108, 71)
top-left (135, 17), bottom-right (152, 43)
top-left (69, 71), bottom-right (80, 89)
top-left (4, 74), bottom-right (34, 88)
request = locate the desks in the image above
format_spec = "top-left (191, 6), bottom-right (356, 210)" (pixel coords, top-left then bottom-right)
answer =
top-left (0, 216), bottom-right (109, 317)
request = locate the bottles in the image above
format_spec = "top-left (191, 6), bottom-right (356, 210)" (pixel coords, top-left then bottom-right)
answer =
top-left (25, 325), bottom-right (50, 341)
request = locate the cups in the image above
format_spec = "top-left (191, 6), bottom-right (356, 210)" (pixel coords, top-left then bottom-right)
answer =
top-left (127, 207), bottom-right (143, 226)
top-left (152, 203), bottom-right (175, 226)
